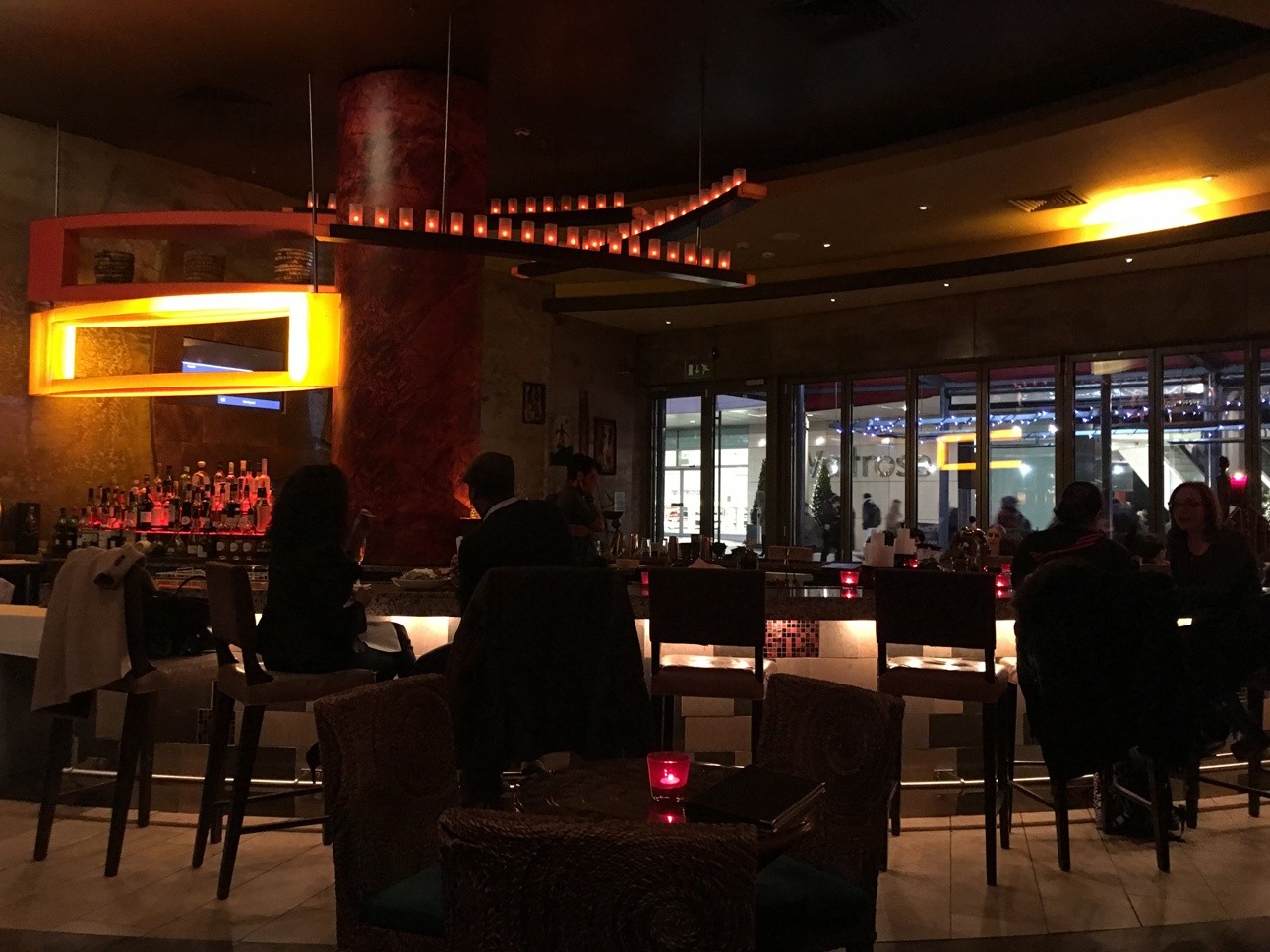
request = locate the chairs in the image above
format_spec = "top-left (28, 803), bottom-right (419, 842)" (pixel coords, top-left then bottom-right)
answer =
top-left (33, 558), bottom-right (1270, 952)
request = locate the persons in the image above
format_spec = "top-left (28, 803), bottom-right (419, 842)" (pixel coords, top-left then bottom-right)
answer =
top-left (995, 495), bottom-right (1031, 529)
top-left (546, 454), bottom-right (604, 560)
top-left (1012, 480), bottom-right (1227, 837)
top-left (939, 508), bottom-right (976, 533)
top-left (253, 465), bottom-right (402, 784)
top-left (1111, 498), bottom-right (1160, 560)
top-left (402, 453), bottom-right (570, 677)
top-left (1143, 482), bottom-right (1270, 771)
top-left (885, 499), bottom-right (902, 528)
top-left (820, 495), bottom-right (854, 562)
top-left (859, 493), bottom-right (881, 555)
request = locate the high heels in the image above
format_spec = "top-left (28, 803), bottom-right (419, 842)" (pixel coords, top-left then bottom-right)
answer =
top-left (306, 742), bottom-right (324, 783)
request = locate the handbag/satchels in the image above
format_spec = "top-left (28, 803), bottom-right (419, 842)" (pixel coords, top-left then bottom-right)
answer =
top-left (1092, 771), bottom-right (1180, 835)
top-left (141, 576), bottom-right (210, 659)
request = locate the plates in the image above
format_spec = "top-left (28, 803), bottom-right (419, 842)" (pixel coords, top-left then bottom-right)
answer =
top-left (391, 577), bottom-right (446, 590)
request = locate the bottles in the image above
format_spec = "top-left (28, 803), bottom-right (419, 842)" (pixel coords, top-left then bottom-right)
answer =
top-left (52, 458), bottom-right (273, 551)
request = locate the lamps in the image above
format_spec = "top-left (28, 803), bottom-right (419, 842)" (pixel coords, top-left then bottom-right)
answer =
top-left (26, 72), bottom-right (348, 398)
top-left (308, 0), bottom-right (767, 290)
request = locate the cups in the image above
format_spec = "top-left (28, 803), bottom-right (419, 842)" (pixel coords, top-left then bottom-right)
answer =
top-left (910, 558), bottom-right (919, 568)
top-left (995, 574), bottom-right (1011, 587)
top-left (840, 567), bottom-right (860, 589)
top-left (647, 751), bottom-right (692, 801)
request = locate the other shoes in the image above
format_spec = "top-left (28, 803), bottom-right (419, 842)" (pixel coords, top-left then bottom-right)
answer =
top-left (1113, 746), bottom-right (1152, 812)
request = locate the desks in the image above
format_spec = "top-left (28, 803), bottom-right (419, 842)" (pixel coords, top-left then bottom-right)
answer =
top-left (821, 562), bottom-right (862, 571)
top-left (502, 758), bottom-right (819, 871)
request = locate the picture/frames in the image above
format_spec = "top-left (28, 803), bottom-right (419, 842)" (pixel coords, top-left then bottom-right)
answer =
top-left (522, 381), bottom-right (547, 425)
top-left (593, 416), bottom-right (617, 476)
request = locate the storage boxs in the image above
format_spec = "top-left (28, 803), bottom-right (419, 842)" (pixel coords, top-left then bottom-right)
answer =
top-left (769, 546), bottom-right (812, 568)
top-left (617, 559), bottom-right (641, 568)
